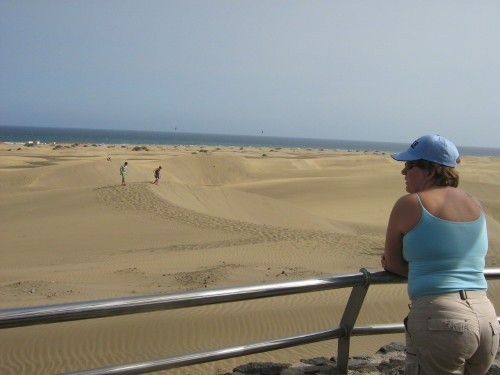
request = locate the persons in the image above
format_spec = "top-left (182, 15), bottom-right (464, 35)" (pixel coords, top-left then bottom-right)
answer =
top-left (381, 134), bottom-right (500, 375)
top-left (120, 161), bottom-right (129, 186)
top-left (153, 166), bottom-right (162, 185)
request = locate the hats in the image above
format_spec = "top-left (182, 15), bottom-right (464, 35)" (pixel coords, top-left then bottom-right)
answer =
top-left (390, 134), bottom-right (460, 168)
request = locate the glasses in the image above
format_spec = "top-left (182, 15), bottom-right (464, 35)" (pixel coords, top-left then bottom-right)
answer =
top-left (404, 162), bottom-right (413, 169)
